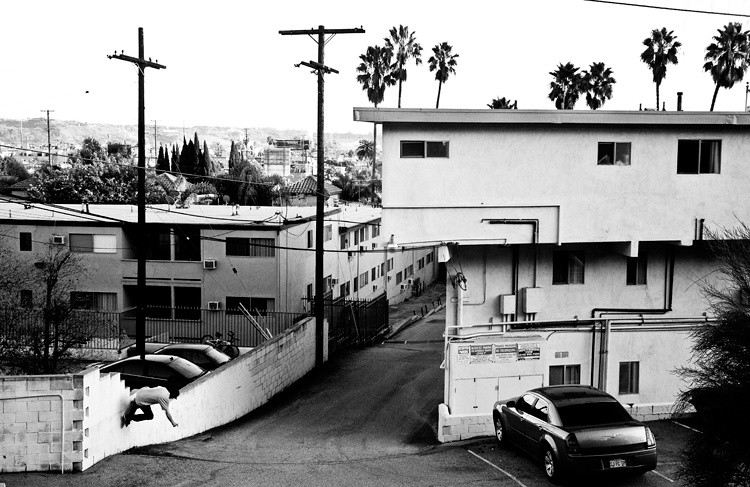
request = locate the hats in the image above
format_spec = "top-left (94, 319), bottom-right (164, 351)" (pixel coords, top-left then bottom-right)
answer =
top-left (171, 388), bottom-right (179, 399)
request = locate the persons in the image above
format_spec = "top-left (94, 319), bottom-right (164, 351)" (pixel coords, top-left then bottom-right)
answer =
top-left (121, 386), bottom-right (180, 428)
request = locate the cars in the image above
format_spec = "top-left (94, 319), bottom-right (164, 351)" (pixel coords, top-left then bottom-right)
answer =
top-left (76, 354), bottom-right (211, 404)
top-left (125, 341), bottom-right (231, 371)
top-left (493, 382), bottom-right (659, 483)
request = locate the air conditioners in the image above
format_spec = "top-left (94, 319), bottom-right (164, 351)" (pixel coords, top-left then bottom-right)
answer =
top-left (204, 259), bottom-right (217, 270)
top-left (53, 235), bottom-right (65, 245)
top-left (208, 300), bottom-right (221, 310)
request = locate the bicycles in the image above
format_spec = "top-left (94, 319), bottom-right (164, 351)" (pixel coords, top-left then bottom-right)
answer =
top-left (200, 329), bottom-right (240, 359)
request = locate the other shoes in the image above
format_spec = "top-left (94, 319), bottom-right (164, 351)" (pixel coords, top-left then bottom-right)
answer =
top-left (125, 414), bottom-right (131, 427)
